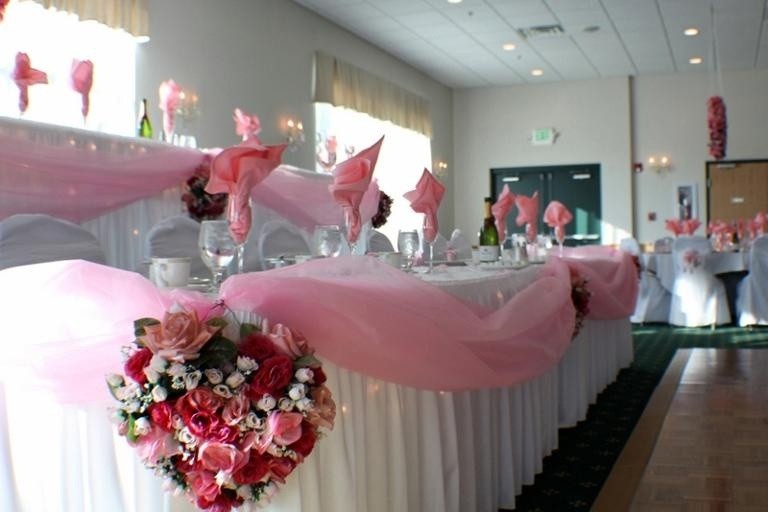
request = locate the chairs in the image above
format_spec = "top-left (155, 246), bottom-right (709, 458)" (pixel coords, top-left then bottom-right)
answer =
top-left (622, 227), bottom-right (768, 332)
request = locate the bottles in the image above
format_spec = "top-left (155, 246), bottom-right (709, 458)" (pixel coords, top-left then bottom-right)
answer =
top-left (731, 223), bottom-right (741, 253)
top-left (478, 196), bottom-right (500, 262)
top-left (139, 98), bottom-right (153, 139)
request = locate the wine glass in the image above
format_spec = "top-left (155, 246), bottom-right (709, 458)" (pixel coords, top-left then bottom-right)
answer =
top-left (341, 204), bottom-right (361, 254)
top-left (226, 192), bottom-right (252, 273)
top-left (197, 219), bottom-right (236, 293)
top-left (163, 112), bottom-right (175, 145)
top-left (81, 93), bottom-right (90, 129)
top-left (422, 213), bottom-right (439, 275)
top-left (397, 229), bottom-right (421, 275)
top-left (18, 85), bottom-right (29, 117)
top-left (500, 221), bottom-right (566, 266)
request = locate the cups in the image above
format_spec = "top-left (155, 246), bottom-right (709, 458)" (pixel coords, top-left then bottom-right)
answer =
top-left (148, 256), bottom-right (192, 288)
top-left (312, 224), bottom-right (341, 256)
top-left (159, 131), bottom-right (196, 149)
